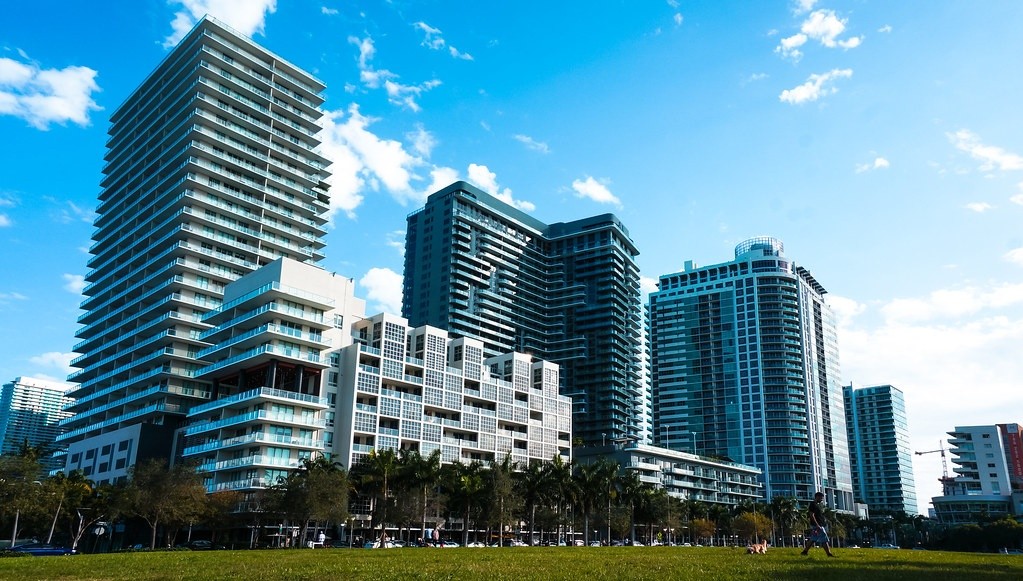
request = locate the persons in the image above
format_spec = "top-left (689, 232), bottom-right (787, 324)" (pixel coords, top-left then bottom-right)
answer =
top-left (318, 531), bottom-right (326, 542)
top-left (433, 528), bottom-right (439, 547)
top-left (379, 531), bottom-right (387, 541)
top-left (800, 492), bottom-right (834, 557)
top-left (425, 527), bottom-right (432, 547)
top-left (440, 537), bottom-right (444, 548)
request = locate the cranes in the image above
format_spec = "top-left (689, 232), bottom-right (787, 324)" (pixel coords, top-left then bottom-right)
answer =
top-left (915, 440), bottom-right (953, 494)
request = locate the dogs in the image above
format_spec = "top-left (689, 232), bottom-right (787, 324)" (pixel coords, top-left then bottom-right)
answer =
top-left (747, 540), bottom-right (768, 554)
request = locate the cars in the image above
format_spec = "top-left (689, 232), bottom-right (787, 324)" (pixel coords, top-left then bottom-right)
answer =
top-left (3, 542), bottom-right (81, 558)
